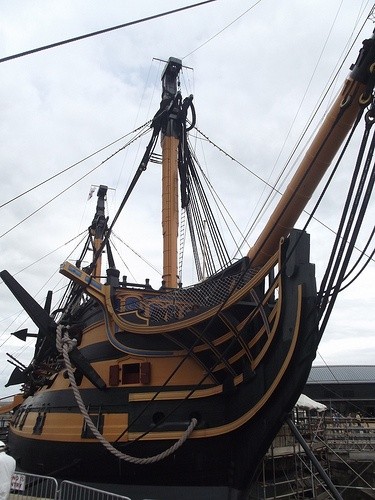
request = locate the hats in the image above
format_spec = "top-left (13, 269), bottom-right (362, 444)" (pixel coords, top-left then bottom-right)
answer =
top-left (0, 440), bottom-right (6, 449)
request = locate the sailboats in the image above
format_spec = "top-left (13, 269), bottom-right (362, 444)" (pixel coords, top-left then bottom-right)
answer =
top-left (0, 25), bottom-right (375, 500)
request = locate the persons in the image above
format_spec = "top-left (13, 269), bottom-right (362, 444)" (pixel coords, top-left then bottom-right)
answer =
top-left (0, 439), bottom-right (18, 499)
